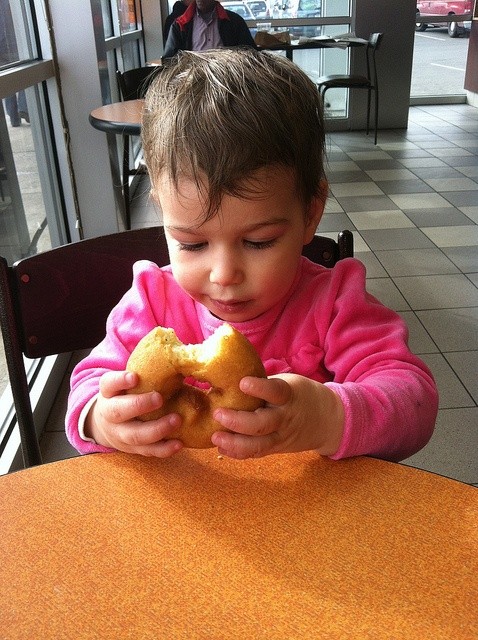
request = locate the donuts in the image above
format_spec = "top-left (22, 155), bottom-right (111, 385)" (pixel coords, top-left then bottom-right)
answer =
top-left (123, 321), bottom-right (264, 448)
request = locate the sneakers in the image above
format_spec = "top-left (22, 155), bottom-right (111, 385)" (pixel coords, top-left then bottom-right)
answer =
top-left (19, 112), bottom-right (30, 123)
top-left (11, 112), bottom-right (20, 126)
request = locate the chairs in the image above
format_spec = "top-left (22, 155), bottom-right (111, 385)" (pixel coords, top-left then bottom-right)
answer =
top-left (0, 227), bottom-right (353, 468)
top-left (314, 31), bottom-right (384, 145)
top-left (116, 67), bottom-right (164, 101)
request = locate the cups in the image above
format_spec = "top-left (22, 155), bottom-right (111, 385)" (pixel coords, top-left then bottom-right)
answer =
top-left (256, 22), bottom-right (272, 33)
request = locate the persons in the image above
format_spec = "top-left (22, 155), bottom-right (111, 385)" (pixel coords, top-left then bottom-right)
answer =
top-left (64, 45), bottom-right (439, 463)
top-left (1, 0), bottom-right (30, 127)
top-left (162, 0), bottom-right (259, 58)
top-left (163, 0), bottom-right (193, 48)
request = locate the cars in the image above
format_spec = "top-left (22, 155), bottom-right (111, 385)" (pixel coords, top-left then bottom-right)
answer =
top-left (220, 1), bottom-right (259, 38)
top-left (415, 0), bottom-right (474, 38)
top-left (281, 0), bottom-right (322, 37)
top-left (246, 0), bottom-right (270, 20)
